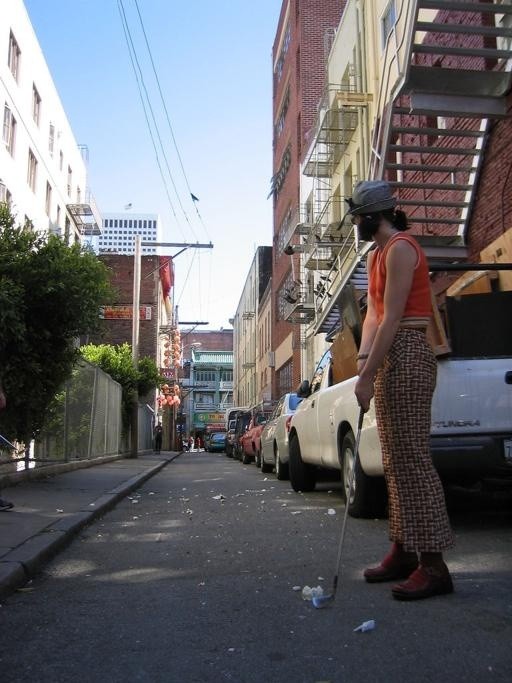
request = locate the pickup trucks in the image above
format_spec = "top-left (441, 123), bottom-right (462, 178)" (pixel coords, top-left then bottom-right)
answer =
top-left (287, 258), bottom-right (512, 518)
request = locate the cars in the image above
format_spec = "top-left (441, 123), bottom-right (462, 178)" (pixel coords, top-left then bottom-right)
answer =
top-left (261, 391), bottom-right (303, 481)
top-left (240, 410), bottom-right (274, 468)
top-left (203, 408), bottom-right (247, 461)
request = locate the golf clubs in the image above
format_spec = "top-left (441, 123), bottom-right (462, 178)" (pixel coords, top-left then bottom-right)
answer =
top-left (313, 407), bottom-right (365, 609)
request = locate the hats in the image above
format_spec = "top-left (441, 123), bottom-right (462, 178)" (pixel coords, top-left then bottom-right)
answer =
top-left (345, 181), bottom-right (398, 217)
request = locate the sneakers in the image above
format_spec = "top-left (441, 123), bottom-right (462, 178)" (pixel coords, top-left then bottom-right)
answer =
top-left (392, 563), bottom-right (456, 599)
top-left (364, 547), bottom-right (419, 582)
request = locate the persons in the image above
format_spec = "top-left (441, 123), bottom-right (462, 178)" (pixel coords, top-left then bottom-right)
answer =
top-left (0, 386), bottom-right (15, 512)
top-left (154, 422), bottom-right (163, 454)
top-left (347, 180), bottom-right (456, 601)
top-left (188, 437), bottom-right (200, 453)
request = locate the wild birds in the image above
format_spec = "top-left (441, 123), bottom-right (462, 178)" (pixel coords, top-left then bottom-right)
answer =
top-left (294, 278), bottom-right (302, 286)
top-left (314, 234), bottom-right (321, 242)
top-left (313, 281), bottom-right (327, 299)
top-left (190, 194), bottom-right (199, 201)
top-left (329, 234), bottom-right (335, 243)
top-left (339, 233), bottom-right (344, 243)
top-left (302, 236), bottom-right (307, 243)
top-left (320, 276), bottom-right (331, 282)
top-left (326, 262), bottom-right (338, 272)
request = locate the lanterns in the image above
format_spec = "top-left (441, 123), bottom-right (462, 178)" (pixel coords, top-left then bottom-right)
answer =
top-left (161, 330), bottom-right (181, 407)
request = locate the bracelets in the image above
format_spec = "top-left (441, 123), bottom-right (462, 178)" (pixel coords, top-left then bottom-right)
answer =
top-left (355, 354), bottom-right (370, 362)
